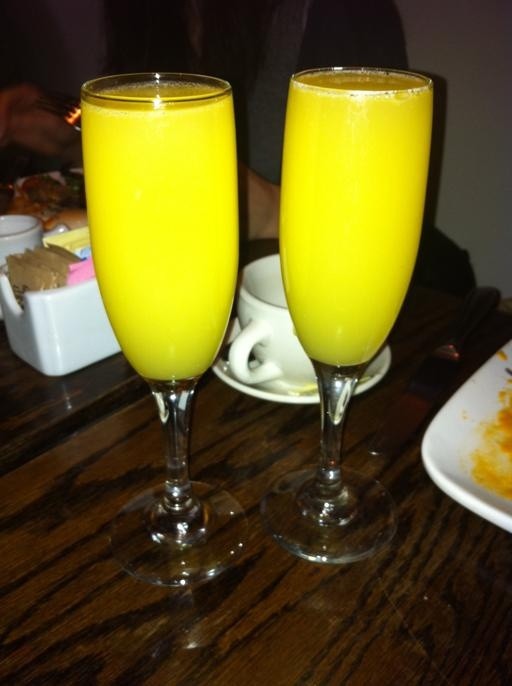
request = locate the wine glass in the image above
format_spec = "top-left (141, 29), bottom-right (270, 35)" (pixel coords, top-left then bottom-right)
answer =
top-left (80, 72), bottom-right (251, 588)
top-left (263, 66), bottom-right (435, 565)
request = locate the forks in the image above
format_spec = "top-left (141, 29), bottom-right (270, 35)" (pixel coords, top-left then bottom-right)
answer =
top-left (36, 94), bottom-right (81, 126)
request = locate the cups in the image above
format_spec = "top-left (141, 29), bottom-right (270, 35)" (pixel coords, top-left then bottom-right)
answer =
top-left (0, 215), bottom-right (71, 267)
top-left (212, 252), bottom-right (392, 405)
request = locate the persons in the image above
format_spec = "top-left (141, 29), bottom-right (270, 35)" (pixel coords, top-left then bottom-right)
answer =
top-left (0, 0), bottom-right (412, 246)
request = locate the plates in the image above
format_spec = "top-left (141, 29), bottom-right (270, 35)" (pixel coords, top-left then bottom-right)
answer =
top-left (214, 316), bottom-right (390, 405)
top-left (420, 340), bottom-right (512, 533)
